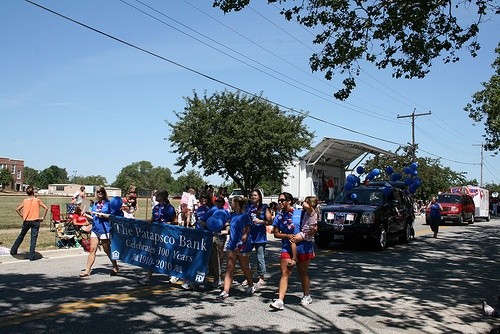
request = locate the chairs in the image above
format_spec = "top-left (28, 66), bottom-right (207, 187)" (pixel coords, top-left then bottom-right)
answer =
top-left (68, 213), bottom-right (89, 240)
top-left (48, 204), bottom-right (66, 233)
top-left (54, 223), bottom-right (74, 248)
top-left (66, 202), bottom-right (79, 214)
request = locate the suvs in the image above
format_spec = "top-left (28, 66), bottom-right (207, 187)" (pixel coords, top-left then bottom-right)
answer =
top-left (425, 193), bottom-right (476, 226)
top-left (317, 180), bottom-right (416, 252)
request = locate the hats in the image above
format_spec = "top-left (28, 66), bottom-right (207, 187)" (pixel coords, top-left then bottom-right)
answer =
top-left (215, 197), bottom-right (225, 204)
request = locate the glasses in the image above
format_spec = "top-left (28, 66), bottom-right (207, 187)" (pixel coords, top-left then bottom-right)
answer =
top-left (278, 199), bottom-right (286, 203)
top-left (97, 191), bottom-right (105, 193)
top-left (200, 196), bottom-right (207, 199)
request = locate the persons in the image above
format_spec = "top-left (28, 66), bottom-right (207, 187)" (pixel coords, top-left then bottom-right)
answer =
top-left (427, 196), bottom-right (443, 237)
top-left (71, 186), bottom-right (86, 207)
top-left (87, 186), bottom-right (321, 311)
top-left (10, 186), bottom-right (48, 261)
top-left (79, 185), bottom-right (120, 277)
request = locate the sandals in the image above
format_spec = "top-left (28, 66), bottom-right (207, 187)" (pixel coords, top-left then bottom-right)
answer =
top-left (287, 257), bottom-right (298, 267)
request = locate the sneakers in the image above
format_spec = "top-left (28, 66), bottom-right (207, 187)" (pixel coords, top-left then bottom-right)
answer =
top-left (300, 294), bottom-right (313, 306)
top-left (269, 299), bottom-right (285, 310)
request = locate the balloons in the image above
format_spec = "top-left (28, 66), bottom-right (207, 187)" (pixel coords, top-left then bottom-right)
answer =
top-left (411, 162), bottom-right (418, 170)
top-left (387, 167), bottom-right (393, 174)
top-left (409, 185), bottom-right (416, 193)
top-left (344, 182), bottom-right (353, 191)
top-left (212, 209), bottom-right (228, 222)
top-left (347, 174), bottom-right (356, 183)
top-left (397, 173), bottom-right (402, 179)
top-left (411, 170), bottom-right (418, 176)
top-left (110, 196), bottom-right (122, 211)
top-left (404, 167), bottom-right (411, 174)
top-left (372, 168), bottom-right (380, 175)
top-left (391, 173), bottom-right (398, 181)
top-left (412, 178), bottom-right (421, 187)
top-left (206, 216), bottom-right (224, 232)
top-left (404, 177), bottom-right (412, 185)
top-left (357, 166), bottom-right (363, 174)
top-left (367, 172), bottom-right (375, 180)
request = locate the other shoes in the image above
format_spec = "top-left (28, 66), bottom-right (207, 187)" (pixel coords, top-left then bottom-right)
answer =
top-left (168, 275), bottom-right (180, 284)
top-left (245, 285), bottom-right (256, 296)
top-left (79, 272), bottom-right (90, 277)
top-left (256, 278), bottom-right (264, 287)
top-left (434, 232), bottom-right (437, 238)
top-left (241, 278), bottom-right (248, 286)
top-left (181, 281), bottom-right (196, 291)
top-left (138, 276), bottom-right (153, 286)
top-left (109, 268), bottom-right (119, 276)
top-left (215, 291), bottom-right (229, 300)
top-left (483, 300), bottom-right (495, 316)
